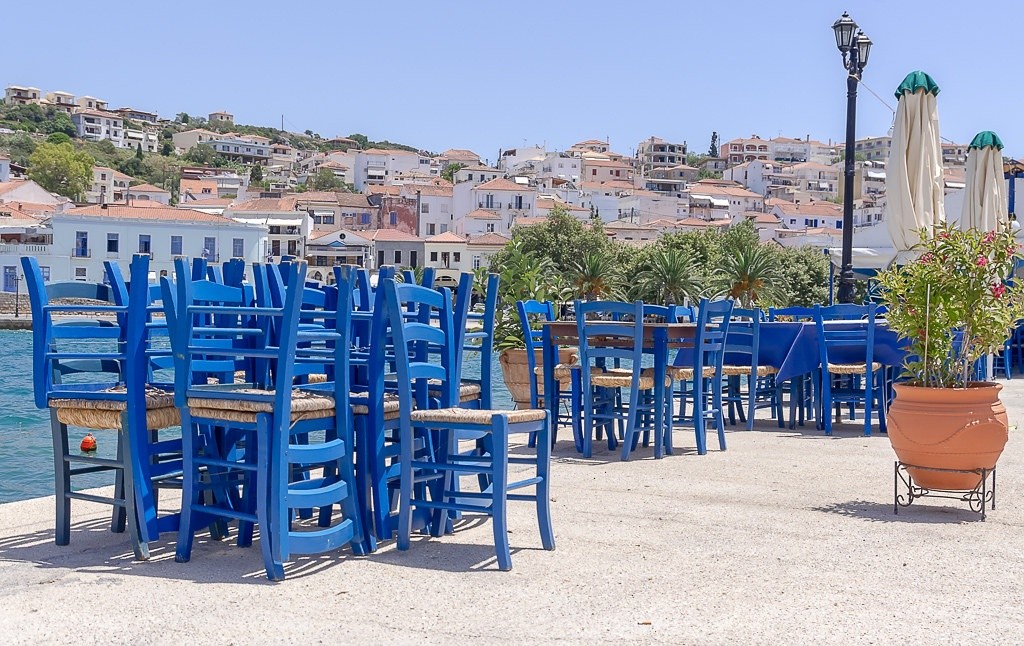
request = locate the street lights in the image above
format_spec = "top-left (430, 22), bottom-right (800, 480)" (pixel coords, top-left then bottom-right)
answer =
top-left (828, 11), bottom-right (873, 303)
top-left (8, 273), bottom-right (25, 317)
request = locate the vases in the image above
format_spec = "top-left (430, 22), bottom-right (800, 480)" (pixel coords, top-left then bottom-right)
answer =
top-left (887, 380), bottom-right (1009, 489)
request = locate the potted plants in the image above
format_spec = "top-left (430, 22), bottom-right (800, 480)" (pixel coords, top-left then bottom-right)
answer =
top-left (471, 202), bottom-right (867, 411)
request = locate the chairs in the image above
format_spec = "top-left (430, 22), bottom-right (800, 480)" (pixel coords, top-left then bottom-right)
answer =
top-left (20, 253), bottom-right (1024, 583)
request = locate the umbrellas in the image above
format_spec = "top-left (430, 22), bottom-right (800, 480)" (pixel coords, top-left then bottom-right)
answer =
top-left (961, 130), bottom-right (1008, 381)
top-left (886, 70), bottom-right (950, 268)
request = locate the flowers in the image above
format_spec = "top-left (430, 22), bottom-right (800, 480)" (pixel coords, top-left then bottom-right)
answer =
top-left (868, 212), bottom-right (1024, 388)
